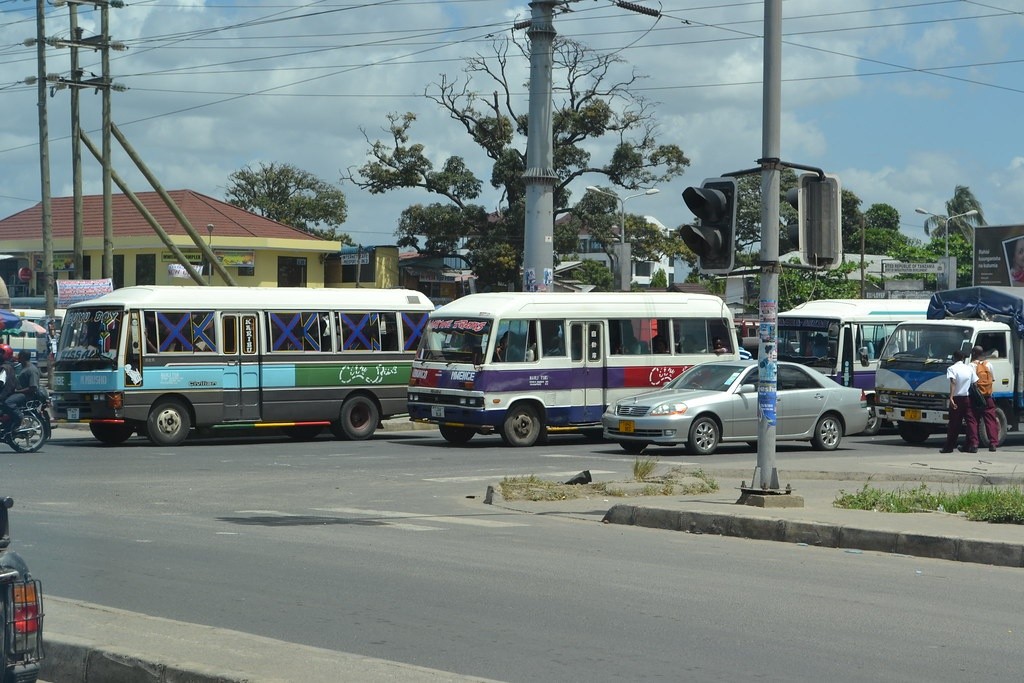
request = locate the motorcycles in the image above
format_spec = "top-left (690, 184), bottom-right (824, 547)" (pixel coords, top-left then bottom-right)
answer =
top-left (0, 400), bottom-right (52, 453)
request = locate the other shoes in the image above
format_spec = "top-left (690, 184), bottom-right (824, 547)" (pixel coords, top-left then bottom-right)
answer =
top-left (989, 445), bottom-right (996, 451)
top-left (958, 446), bottom-right (970, 452)
top-left (970, 447), bottom-right (977, 453)
top-left (939, 447), bottom-right (953, 453)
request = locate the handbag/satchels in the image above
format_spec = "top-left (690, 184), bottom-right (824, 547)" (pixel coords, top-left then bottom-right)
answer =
top-left (969, 386), bottom-right (987, 413)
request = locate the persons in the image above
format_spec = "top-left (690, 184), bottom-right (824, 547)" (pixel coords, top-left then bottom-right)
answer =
top-left (957, 346), bottom-right (999, 451)
top-left (496, 331), bottom-right (521, 362)
top-left (939, 350), bottom-right (979, 453)
top-left (737, 337), bottom-right (753, 361)
top-left (0, 344), bottom-right (39, 429)
top-left (809, 334), bottom-right (828, 357)
top-left (981, 335), bottom-right (998, 358)
top-left (712, 336), bottom-right (728, 353)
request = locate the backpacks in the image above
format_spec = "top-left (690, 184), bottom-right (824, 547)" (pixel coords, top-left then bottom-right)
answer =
top-left (971, 360), bottom-right (993, 396)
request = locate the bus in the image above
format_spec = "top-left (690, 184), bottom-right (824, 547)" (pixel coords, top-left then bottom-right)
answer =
top-left (734, 298), bottom-right (931, 436)
top-left (50, 285), bottom-right (436, 447)
top-left (1, 308), bottom-right (82, 368)
top-left (408, 292), bottom-right (738, 447)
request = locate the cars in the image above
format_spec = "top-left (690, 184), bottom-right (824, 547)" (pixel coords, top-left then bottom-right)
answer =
top-left (601, 360), bottom-right (870, 456)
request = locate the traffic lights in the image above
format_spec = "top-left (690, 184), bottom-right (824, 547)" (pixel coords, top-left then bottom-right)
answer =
top-left (786, 173), bottom-right (843, 270)
top-left (679, 177), bottom-right (737, 273)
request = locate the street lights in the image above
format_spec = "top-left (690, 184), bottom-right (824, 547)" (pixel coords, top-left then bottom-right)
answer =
top-left (585, 185), bottom-right (660, 292)
top-left (914, 207), bottom-right (978, 292)
top-left (206, 223), bottom-right (214, 284)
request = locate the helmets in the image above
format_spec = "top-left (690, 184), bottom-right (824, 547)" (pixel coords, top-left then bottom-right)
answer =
top-left (0, 344), bottom-right (14, 362)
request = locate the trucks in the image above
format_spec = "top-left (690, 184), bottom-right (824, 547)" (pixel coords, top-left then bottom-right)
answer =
top-left (858, 286), bottom-right (1024, 447)
